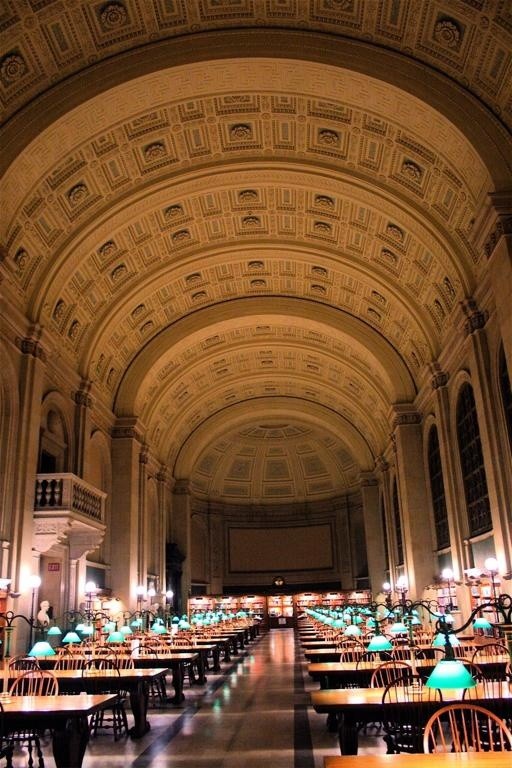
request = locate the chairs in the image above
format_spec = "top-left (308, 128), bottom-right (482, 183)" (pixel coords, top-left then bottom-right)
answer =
top-left (0, 622), bottom-right (238, 768)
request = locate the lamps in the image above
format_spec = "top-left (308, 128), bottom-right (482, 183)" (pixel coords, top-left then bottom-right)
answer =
top-left (0, 609), bottom-right (167, 658)
top-left (332, 593), bottom-right (512, 689)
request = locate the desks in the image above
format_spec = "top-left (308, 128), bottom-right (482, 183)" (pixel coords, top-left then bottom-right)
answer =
top-left (295, 618), bottom-right (512, 768)
top-left (176, 621), bottom-right (260, 658)
